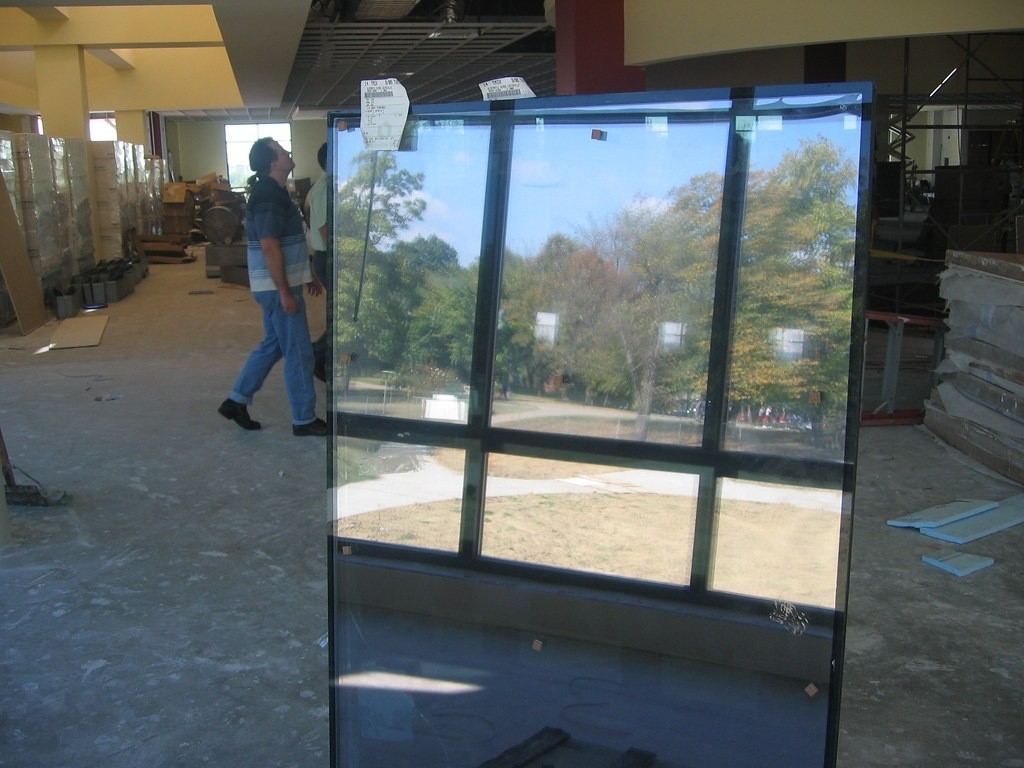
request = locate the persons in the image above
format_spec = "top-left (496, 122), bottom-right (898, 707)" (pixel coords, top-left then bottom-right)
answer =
top-left (309, 142), bottom-right (327, 382)
top-left (498, 371), bottom-right (509, 400)
top-left (216, 137), bottom-right (327, 436)
top-left (758, 407), bottom-right (765, 425)
top-left (763, 406), bottom-right (772, 428)
top-left (781, 408), bottom-right (791, 430)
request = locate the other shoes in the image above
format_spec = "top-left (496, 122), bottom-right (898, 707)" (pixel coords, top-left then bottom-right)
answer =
top-left (316, 368), bottom-right (326, 383)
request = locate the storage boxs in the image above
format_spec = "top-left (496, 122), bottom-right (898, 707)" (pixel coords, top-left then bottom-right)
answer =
top-left (53, 173), bottom-right (229, 318)
top-left (285, 176), bottom-right (310, 191)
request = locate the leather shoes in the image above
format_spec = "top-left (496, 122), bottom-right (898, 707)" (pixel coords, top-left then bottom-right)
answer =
top-left (293, 417), bottom-right (329, 437)
top-left (218, 397), bottom-right (261, 430)
